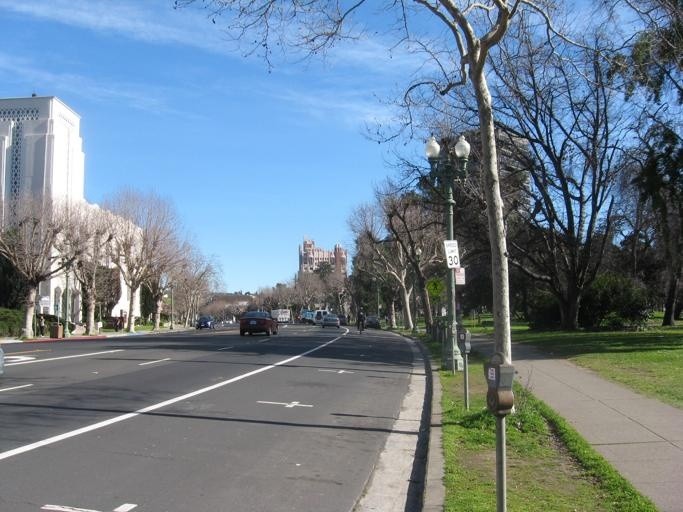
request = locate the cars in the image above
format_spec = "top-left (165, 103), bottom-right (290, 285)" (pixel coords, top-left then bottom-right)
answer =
top-left (365, 316), bottom-right (380, 329)
top-left (240, 311), bottom-right (278, 335)
top-left (300, 309), bottom-right (347, 328)
top-left (195, 316), bottom-right (215, 329)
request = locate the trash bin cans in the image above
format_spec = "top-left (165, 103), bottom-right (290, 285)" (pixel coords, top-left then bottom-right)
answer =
top-left (50, 322), bottom-right (63, 339)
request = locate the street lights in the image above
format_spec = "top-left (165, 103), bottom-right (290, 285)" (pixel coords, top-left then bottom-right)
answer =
top-left (373, 276), bottom-right (380, 316)
top-left (61, 239), bottom-right (75, 338)
top-left (425, 133), bottom-right (472, 367)
top-left (413, 246), bottom-right (421, 333)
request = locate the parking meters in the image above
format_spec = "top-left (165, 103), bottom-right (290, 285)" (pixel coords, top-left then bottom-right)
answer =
top-left (482, 352), bottom-right (516, 512)
top-left (448, 320), bottom-right (457, 376)
top-left (456, 328), bottom-right (472, 413)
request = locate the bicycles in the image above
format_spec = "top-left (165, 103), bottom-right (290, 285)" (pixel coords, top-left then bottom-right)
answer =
top-left (359, 320), bottom-right (364, 334)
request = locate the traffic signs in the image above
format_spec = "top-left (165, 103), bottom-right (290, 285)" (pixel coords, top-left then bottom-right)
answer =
top-left (444, 240), bottom-right (460, 268)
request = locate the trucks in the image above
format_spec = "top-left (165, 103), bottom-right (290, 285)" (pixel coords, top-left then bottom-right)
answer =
top-left (271, 309), bottom-right (290, 322)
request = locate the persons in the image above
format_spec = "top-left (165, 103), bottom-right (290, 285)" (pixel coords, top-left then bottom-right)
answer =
top-left (39, 315), bottom-right (45, 337)
top-left (357, 306), bottom-right (366, 331)
